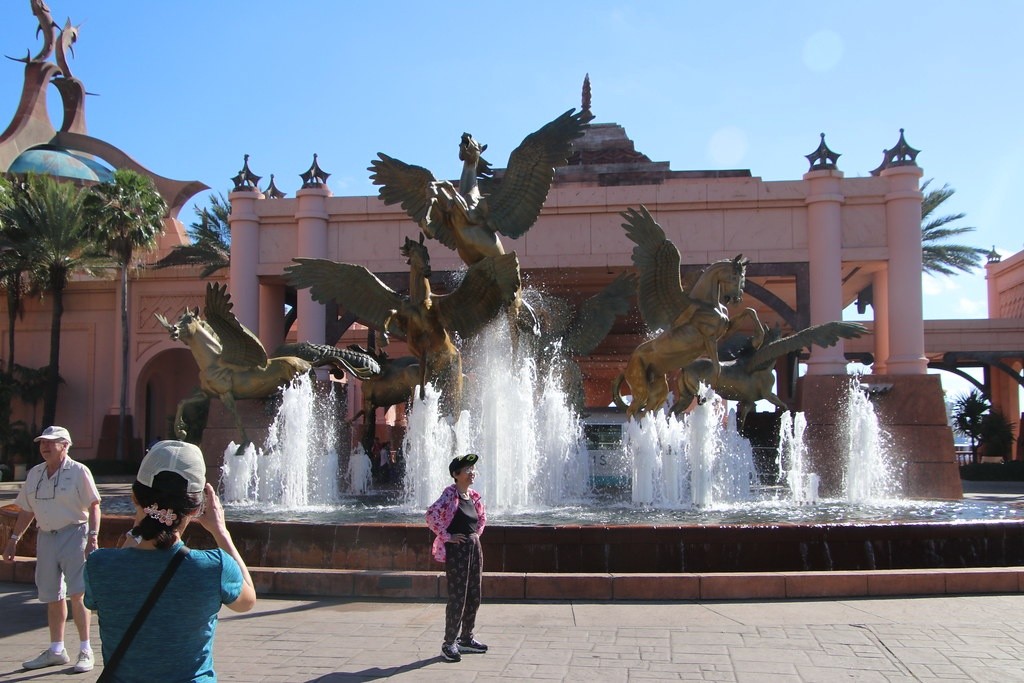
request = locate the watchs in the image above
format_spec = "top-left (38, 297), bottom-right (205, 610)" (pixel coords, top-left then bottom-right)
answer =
top-left (87, 530), bottom-right (99, 535)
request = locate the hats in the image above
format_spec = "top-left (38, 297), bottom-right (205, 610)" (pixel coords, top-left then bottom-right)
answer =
top-left (136, 439), bottom-right (208, 494)
top-left (34, 425), bottom-right (73, 445)
top-left (448, 453), bottom-right (479, 474)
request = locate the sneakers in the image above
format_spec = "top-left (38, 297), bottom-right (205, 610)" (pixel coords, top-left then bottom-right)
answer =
top-left (459, 639), bottom-right (489, 654)
top-left (440, 643), bottom-right (463, 661)
top-left (73, 649), bottom-right (96, 671)
top-left (22, 649), bottom-right (70, 670)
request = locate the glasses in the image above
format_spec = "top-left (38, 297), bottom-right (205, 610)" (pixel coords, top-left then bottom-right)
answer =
top-left (34, 477), bottom-right (58, 501)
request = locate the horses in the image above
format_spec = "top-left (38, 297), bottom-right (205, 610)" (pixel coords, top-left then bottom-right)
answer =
top-left (345, 130), bottom-right (586, 454)
top-left (612, 253), bottom-right (764, 418)
top-left (168, 304), bottom-right (311, 440)
top-left (668, 321), bottom-right (790, 432)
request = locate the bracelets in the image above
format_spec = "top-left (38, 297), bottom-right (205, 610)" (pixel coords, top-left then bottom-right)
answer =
top-left (127, 528), bottom-right (143, 544)
top-left (10, 534), bottom-right (21, 542)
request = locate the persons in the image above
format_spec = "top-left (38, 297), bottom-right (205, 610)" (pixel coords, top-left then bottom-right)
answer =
top-left (424, 453), bottom-right (488, 662)
top-left (83, 440), bottom-right (257, 683)
top-left (2, 426), bottom-right (101, 672)
